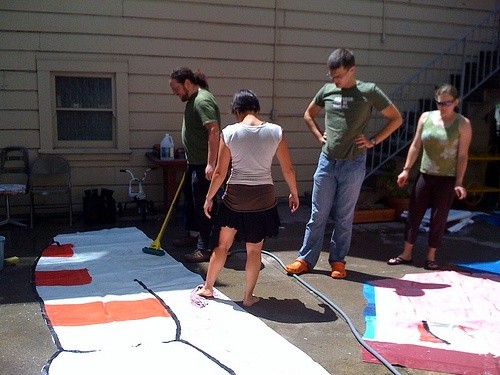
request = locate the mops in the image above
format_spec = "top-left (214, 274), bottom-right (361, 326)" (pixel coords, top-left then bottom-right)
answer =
top-left (142, 172), bottom-right (187, 256)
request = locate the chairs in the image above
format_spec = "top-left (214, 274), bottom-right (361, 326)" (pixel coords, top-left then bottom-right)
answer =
top-left (0, 145), bottom-right (31, 228)
top-left (29, 154), bottom-right (75, 227)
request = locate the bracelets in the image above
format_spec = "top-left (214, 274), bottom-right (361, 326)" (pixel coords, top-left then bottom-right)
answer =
top-left (403, 167), bottom-right (410, 170)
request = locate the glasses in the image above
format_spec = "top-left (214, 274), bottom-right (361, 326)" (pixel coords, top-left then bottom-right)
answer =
top-left (435, 97), bottom-right (456, 106)
top-left (327, 68), bottom-right (350, 81)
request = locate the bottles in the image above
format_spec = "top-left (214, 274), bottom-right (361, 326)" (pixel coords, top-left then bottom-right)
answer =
top-left (160, 134), bottom-right (175, 160)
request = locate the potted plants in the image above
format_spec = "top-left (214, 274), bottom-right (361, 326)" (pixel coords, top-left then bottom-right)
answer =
top-left (380, 174), bottom-right (409, 221)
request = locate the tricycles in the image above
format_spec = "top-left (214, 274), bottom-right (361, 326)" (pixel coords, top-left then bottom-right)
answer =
top-left (119, 168), bottom-right (158, 219)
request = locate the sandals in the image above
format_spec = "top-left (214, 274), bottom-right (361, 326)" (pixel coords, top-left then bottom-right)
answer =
top-left (285, 258), bottom-right (309, 274)
top-left (330, 261), bottom-right (347, 278)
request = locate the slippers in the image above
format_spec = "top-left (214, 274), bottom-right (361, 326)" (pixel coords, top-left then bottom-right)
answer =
top-left (197, 288), bottom-right (213, 298)
top-left (424, 258), bottom-right (437, 269)
top-left (388, 256), bottom-right (412, 264)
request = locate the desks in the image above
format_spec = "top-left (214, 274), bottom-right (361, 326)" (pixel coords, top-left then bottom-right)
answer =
top-left (145, 153), bottom-right (189, 228)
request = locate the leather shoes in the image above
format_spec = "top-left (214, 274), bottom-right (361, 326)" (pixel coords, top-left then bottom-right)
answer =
top-left (173, 235), bottom-right (197, 246)
top-left (184, 249), bottom-right (209, 262)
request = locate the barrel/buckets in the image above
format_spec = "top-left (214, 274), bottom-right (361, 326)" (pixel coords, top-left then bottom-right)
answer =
top-left (390, 197), bottom-right (410, 223)
top-left (82, 188), bottom-right (100, 227)
top-left (0, 235), bottom-right (6, 271)
top-left (97, 187), bottom-right (118, 226)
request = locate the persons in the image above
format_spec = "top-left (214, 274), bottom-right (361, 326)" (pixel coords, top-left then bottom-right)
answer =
top-left (285, 48), bottom-right (402, 278)
top-left (387, 84), bottom-right (471, 269)
top-left (169, 66), bottom-right (231, 261)
top-left (196, 89), bottom-right (300, 307)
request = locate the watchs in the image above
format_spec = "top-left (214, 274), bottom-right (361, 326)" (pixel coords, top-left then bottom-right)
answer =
top-left (370, 136), bottom-right (377, 146)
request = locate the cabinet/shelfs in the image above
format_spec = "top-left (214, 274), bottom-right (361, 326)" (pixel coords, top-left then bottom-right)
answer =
top-left (468, 153), bottom-right (500, 212)
